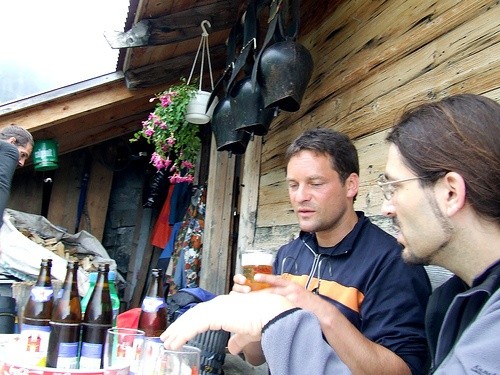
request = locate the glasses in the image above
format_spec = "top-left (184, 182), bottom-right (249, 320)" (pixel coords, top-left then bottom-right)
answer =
top-left (377, 171), bottom-right (447, 201)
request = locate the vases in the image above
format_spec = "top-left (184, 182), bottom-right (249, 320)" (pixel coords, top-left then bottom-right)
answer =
top-left (184, 91), bottom-right (219, 125)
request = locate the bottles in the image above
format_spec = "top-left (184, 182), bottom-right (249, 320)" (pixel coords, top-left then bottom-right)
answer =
top-left (130, 269), bottom-right (168, 375)
top-left (20, 259), bottom-right (55, 367)
top-left (78, 263), bottom-right (120, 370)
top-left (45, 261), bottom-right (83, 370)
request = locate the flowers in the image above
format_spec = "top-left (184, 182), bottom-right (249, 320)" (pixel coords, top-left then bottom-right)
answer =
top-left (128, 76), bottom-right (202, 184)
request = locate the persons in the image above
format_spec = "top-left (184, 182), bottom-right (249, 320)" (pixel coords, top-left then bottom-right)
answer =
top-left (231, 130), bottom-right (432, 375)
top-left (0, 126), bottom-right (34, 229)
top-left (160, 93), bottom-right (500, 375)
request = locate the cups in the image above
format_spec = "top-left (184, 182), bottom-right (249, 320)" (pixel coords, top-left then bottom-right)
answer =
top-left (154, 344), bottom-right (202, 375)
top-left (241, 247), bottom-right (275, 291)
top-left (103, 328), bottom-right (146, 375)
top-left (136, 339), bottom-right (182, 375)
